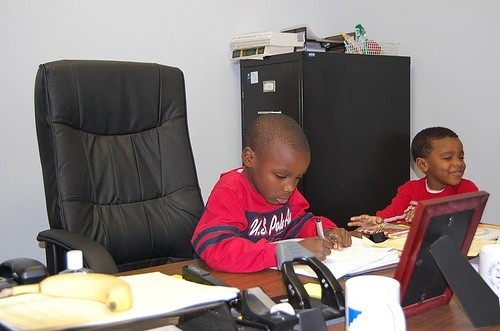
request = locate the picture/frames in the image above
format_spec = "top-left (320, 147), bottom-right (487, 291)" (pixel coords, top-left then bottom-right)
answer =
top-left (394, 190), bottom-right (500, 328)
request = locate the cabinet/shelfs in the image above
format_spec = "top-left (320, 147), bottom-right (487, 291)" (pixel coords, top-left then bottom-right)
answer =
top-left (240, 51), bottom-right (411, 234)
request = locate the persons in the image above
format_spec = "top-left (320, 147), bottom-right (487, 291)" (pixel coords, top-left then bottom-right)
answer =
top-left (347, 127), bottom-right (479, 231)
top-left (191, 113), bottom-right (352, 273)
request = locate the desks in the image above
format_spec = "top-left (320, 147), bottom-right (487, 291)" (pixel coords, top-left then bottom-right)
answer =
top-left (0, 221), bottom-right (500, 331)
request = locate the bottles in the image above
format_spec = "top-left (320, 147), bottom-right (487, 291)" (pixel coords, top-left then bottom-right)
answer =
top-left (58, 250), bottom-right (93, 275)
top-left (340, 31), bottom-right (355, 43)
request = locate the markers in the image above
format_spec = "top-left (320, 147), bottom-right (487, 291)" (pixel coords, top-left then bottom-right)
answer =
top-left (315, 216), bottom-right (325, 240)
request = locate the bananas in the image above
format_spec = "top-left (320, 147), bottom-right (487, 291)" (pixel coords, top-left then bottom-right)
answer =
top-left (0, 272), bottom-right (133, 313)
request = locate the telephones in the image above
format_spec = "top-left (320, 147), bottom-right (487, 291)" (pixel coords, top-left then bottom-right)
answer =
top-left (0, 257), bottom-right (49, 292)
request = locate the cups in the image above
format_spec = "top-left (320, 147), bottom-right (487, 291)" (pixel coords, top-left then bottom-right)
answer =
top-left (478, 243), bottom-right (499, 300)
top-left (345, 275), bottom-right (407, 331)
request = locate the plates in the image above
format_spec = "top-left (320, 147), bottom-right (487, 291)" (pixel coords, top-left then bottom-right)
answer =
top-left (0, 271), bottom-right (241, 331)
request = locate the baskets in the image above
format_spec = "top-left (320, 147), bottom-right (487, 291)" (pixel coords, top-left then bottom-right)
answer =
top-left (345, 40), bottom-right (400, 56)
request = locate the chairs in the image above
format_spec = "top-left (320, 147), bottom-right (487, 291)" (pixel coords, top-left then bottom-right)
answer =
top-left (33, 59), bottom-right (205, 274)
